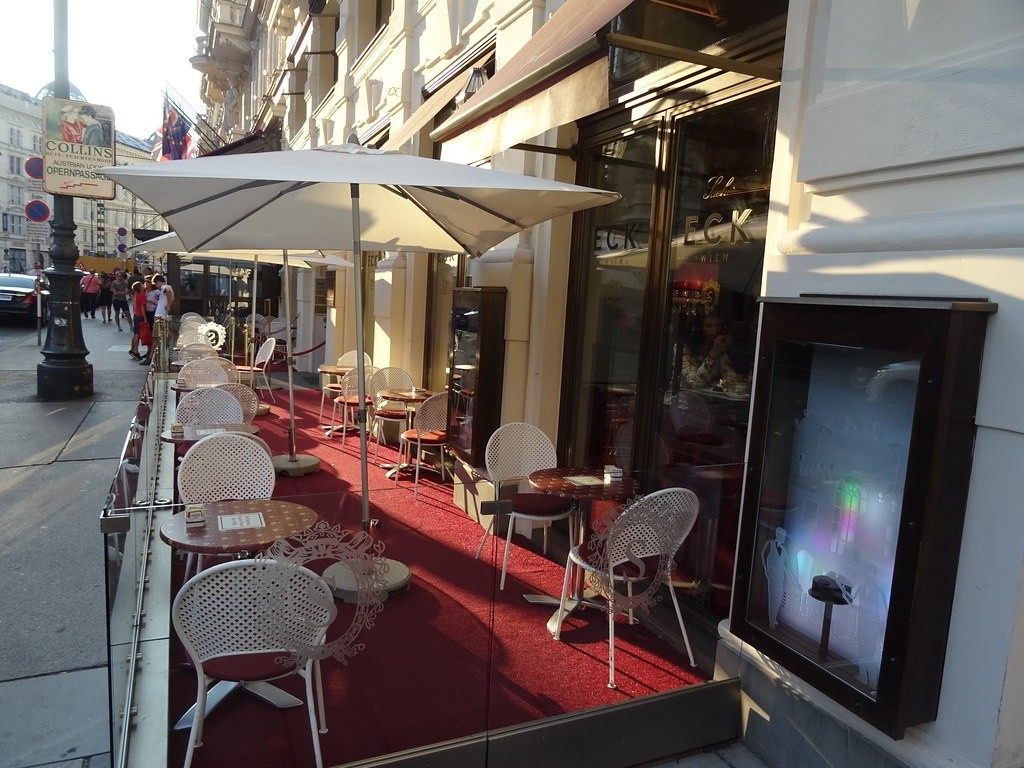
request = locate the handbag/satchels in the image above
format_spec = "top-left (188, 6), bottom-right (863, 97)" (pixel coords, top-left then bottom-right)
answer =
top-left (140, 321), bottom-right (154, 345)
top-left (81, 292), bottom-right (86, 311)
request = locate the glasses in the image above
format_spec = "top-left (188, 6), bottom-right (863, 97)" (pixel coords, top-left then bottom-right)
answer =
top-left (144, 281), bottom-right (151, 283)
top-left (117, 275), bottom-right (123, 278)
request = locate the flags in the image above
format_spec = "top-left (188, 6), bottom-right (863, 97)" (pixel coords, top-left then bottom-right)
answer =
top-left (146, 94), bottom-right (199, 162)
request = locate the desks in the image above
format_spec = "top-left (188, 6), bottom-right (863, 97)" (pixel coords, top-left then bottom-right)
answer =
top-left (172, 346), bottom-right (222, 352)
top-left (158, 500), bottom-right (318, 730)
top-left (171, 381), bottom-right (224, 408)
top-left (318, 366), bottom-right (361, 436)
top-left (523, 467), bottom-right (639, 635)
top-left (172, 359), bottom-right (197, 371)
top-left (160, 424), bottom-right (259, 443)
top-left (679, 387), bottom-right (751, 426)
top-left (379, 388), bottom-right (441, 477)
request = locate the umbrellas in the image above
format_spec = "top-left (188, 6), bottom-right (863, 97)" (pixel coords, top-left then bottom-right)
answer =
top-left (123, 232), bottom-right (362, 461)
top-left (91, 143), bottom-right (624, 575)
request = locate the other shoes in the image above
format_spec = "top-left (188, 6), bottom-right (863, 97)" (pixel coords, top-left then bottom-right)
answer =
top-left (130, 327), bottom-right (134, 331)
top-left (84, 311), bottom-right (88, 318)
top-left (122, 314), bottom-right (124, 318)
top-left (90, 313), bottom-right (96, 318)
top-left (118, 328), bottom-right (122, 332)
top-left (141, 353), bottom-right (149, 357)
top-left (103, 320), bottom-right (106, 323)
top-left (108, 319), bottom-right (112, 322)
top-left (139, 357), bottom-right (151, 365)
top-left (128, 350), bottom-right (143, 360)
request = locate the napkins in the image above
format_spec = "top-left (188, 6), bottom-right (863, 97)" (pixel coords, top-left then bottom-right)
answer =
top-left (605, 465), bottom-right (622, 482)
top-left (186, 503), bottom-right (206, 528)
top-left (412, 387), bottom-right (426, 395)
top-left (170, 425), bottom-right (185, 436)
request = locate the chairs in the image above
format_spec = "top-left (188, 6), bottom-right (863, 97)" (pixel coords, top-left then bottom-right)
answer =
top-left (160, 311), bottom-right (449, 768)
top-left (475, 422), bottom-right (699, 688)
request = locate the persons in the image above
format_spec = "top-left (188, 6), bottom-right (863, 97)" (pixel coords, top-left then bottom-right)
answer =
top-left (3, 261), bottom-right (176, 365)
top-left (663, 308), bottom-right (745, 439)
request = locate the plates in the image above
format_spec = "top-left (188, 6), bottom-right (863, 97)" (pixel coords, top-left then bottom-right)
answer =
top-left (701, 386), bottom-right (727, 395)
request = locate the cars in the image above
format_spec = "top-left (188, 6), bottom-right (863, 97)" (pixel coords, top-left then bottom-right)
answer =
top-left (0, 270), bottom-right (54, 328)
